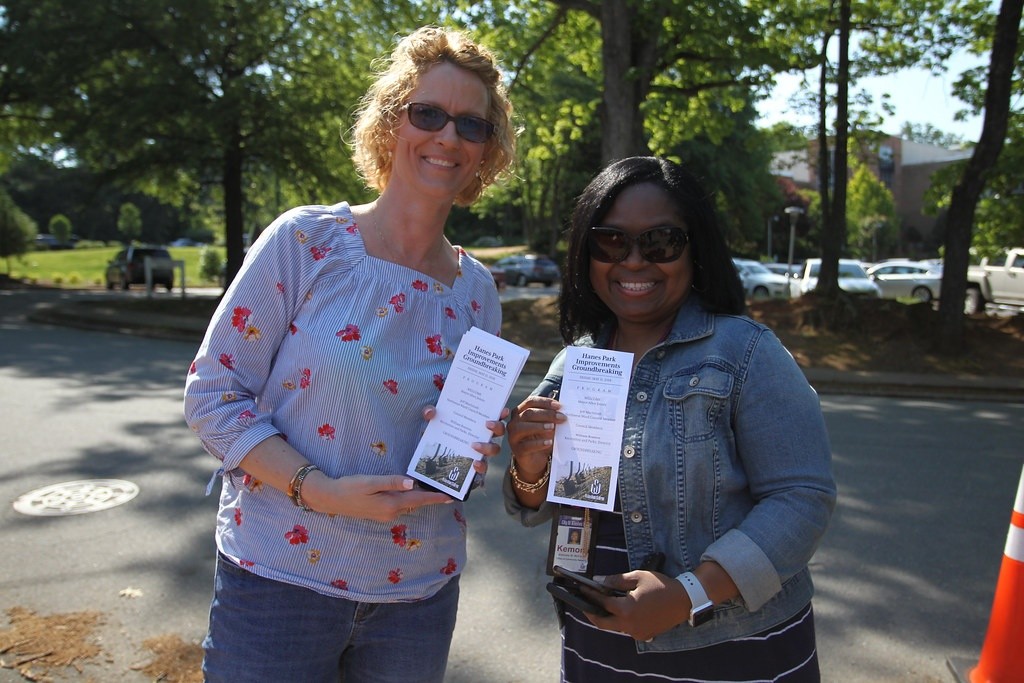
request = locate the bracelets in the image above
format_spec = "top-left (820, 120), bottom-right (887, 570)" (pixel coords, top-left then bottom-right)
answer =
top-left (510, 454), bottom-right (552, 493)
top-left (287, 464), bottom-right (320, 513)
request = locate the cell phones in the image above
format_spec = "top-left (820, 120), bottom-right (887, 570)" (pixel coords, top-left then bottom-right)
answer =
top-left (554, 565), bottom-right (628, 599)
top-left (546, 583), bottom-right (610, 616)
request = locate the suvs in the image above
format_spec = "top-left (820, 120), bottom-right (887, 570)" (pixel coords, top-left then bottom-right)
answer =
top-left (105, 245), bottom-right (175, 291)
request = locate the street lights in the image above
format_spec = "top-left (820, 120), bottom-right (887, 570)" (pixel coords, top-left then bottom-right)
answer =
top-left (784, 205), bottom-right (805, 278)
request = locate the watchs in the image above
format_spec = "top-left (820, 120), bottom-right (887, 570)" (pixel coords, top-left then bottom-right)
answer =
top-left (674, 572), bottom-right (714, 628)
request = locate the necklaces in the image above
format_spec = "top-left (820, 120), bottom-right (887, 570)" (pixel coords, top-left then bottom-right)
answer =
top-left (371, 203), bottom-right (444, 275)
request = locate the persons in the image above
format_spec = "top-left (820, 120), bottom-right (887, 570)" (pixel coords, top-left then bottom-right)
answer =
top-left (568, 529), bottom-right (580, 545)
top-left (502, 157), bottom-right (838, 683)
top-left (184, 26), bottom-right (517, 683)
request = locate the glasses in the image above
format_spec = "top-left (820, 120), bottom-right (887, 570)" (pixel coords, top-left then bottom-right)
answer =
top-left (398, 101), bottom-right (496, 145)
top-left (587, 223), bottom-right (694, 264)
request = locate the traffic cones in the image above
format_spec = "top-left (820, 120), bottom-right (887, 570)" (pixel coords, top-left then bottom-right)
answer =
top-left (947, 458), bottom-right (1024, 683)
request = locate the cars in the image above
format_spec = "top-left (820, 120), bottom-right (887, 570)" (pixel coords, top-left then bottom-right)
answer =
top-left (491, 254), bottom-right (560, 287)
top-left (726, 247), bottom-right (1024, 316)
